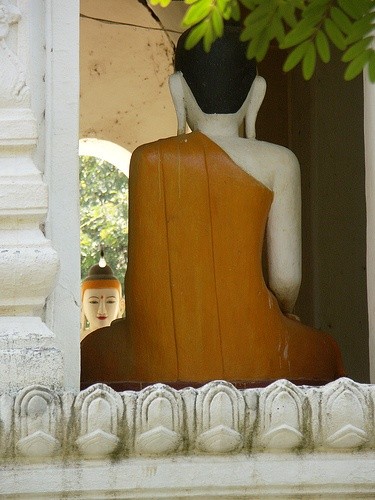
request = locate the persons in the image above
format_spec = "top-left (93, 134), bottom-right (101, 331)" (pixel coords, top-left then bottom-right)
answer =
top-left (81, 264), bottom-right (122, 339)
top-left (79, 18), bottom-right (344, 388)
